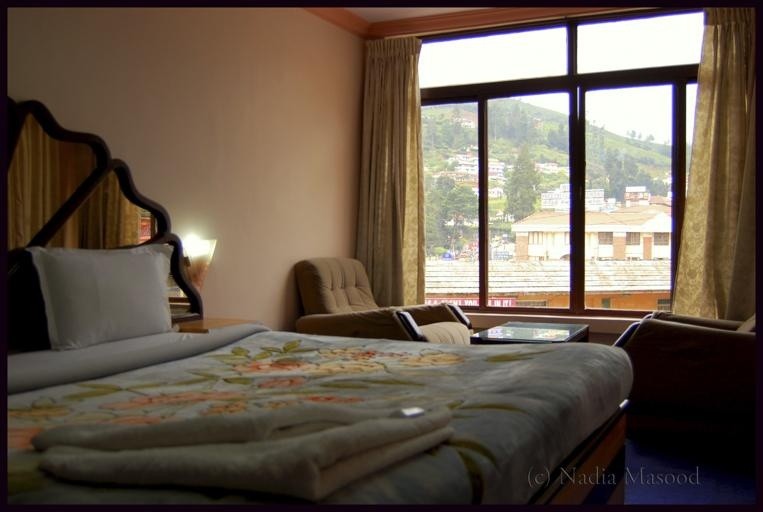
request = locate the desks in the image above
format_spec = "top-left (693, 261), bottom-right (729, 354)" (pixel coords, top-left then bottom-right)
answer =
top-left (470, 321), bottom-right (590, 344)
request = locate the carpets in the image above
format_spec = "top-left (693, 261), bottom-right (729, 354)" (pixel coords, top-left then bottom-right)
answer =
top-left (580, 425), bottom-right (756, 505)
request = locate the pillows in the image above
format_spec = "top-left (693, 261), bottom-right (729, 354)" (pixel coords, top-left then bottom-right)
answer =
top-left (25, 243), bottom-right (177, 351)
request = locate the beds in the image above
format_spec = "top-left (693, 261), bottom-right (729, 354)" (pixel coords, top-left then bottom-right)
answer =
top-left (3, 98), bottom-right (634, 504)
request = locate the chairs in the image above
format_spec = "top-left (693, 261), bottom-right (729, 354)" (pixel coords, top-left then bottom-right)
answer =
top-left (607, 311), bottom-right (757, 419)
top-left (295, 256), bottom-right (475, 345)
